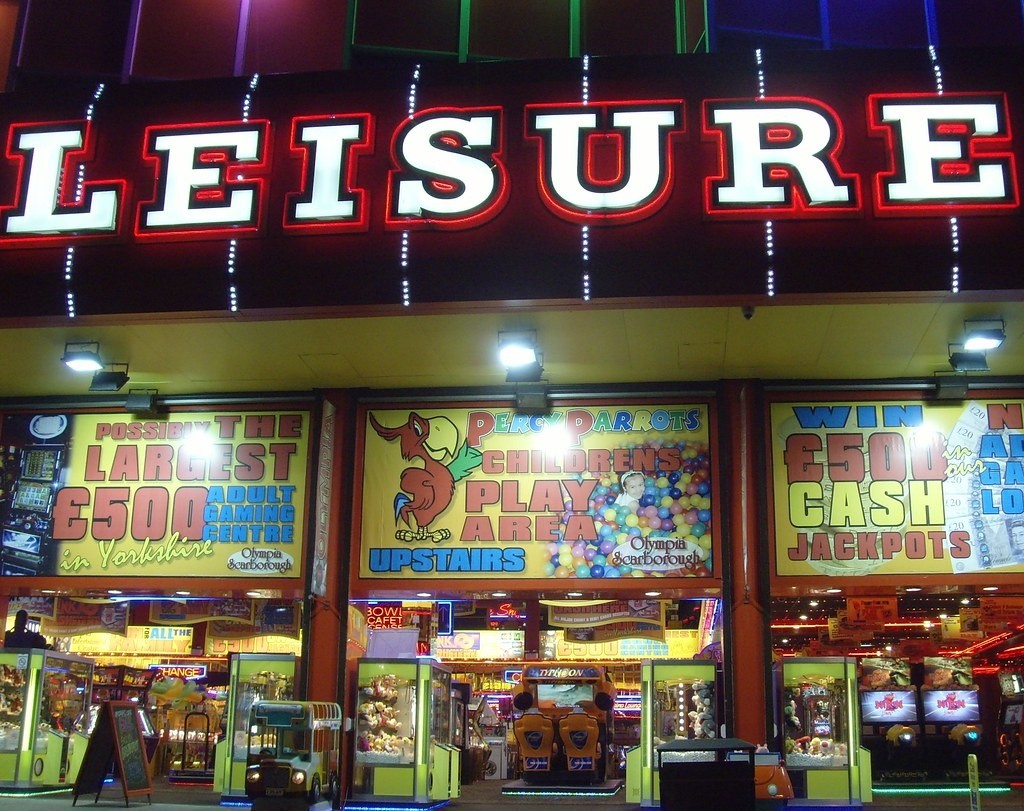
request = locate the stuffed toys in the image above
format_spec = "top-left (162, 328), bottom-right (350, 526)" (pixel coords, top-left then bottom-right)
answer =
top-left (785, 736), bottom-right (847, 756)
top-left (265, 671), bottom-right (294, 700)
top-left (688, 681), bottom-right (715, 737)
top-left (784, 706), bottom-right (803, 732)
top-left (756, 744), bottom-right (769, 753)
top-left (0, 664), bottom-right (23, 716)
top-left (360, 674), bottom-right (415, 753)
top-left (246, 733), bottom-right (276, 746)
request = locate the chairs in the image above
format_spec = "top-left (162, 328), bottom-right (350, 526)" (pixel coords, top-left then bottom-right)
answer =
top-left (513, 711), bottom-right (557, 772)
top-left (559, 712), bottom-right (602, 772)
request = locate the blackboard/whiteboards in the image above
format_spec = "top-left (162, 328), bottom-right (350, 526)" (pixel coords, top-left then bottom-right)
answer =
top-left (71, 701), bottom-right (154, 796)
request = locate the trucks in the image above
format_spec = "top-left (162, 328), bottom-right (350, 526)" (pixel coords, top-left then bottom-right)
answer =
top-left (657, 739), bottom-right (794, 811)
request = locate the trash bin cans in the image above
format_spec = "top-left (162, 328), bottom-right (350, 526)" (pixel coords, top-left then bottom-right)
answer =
top-left (656, 740), bottom-right (758, 811)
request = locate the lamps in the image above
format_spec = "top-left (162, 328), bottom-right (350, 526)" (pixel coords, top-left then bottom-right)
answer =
top-left (60, 342), bottom-right (105, 371)
top-left (515, 378), bottom-right (549, 408)
top-left (505, 353), bottom-right (544, 382)
top-left (963, 319), bottom-right (1006, 351)
top-left (933, 369), bottom-right (970, 399)
top-left (497, 329), bottom-right (544, 366)
top-left (948, 343), bottom-right (991, 372)
top-left (89, 362), bottom-right (130, 392)
top-left (125, 389), bottom-right (158, 411)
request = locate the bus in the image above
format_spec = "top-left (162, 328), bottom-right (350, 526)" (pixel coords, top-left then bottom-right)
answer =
top-left (510, 663), bottom-right (617, 785)
top-left (245, 700), bottom-right (342, 800)
